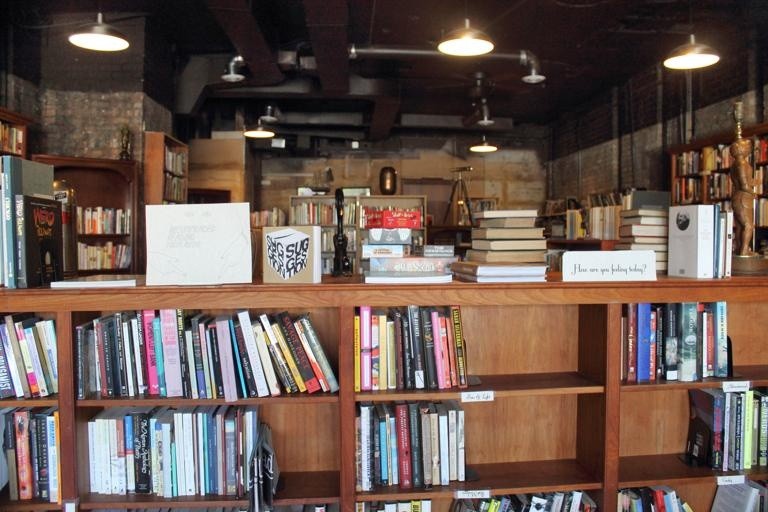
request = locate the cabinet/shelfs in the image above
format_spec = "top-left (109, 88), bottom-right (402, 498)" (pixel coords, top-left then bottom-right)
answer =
top-left (665, 120), bottom-right (768, 259)
top-left (33, 152), bottom-right (140, 275)
top-left (1, 107), bottom-right (32, 160)
top-left (289, 195), bottom-right (428, 274)
top-left (191, 138), bottom-right (250, 203)
top-left (143, 131), bottom-right (191, 206)
top-left (0, 271), bottom-right (768, 512)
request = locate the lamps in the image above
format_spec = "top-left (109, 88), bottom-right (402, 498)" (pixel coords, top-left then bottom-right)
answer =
top-left (436, 19), bottom-right (494, 57)
top-left (67, 12), bottom-right (130, 53)
top-left (469, 138), bottom-right (498, 153)
top-left (243, 118), bottom-right (276, 140)
top-left (663, 34), bottom-right (720, 69)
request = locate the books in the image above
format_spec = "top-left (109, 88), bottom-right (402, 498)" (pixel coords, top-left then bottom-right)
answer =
top-left (685, 389), bottom-right (767, 471)
top-left (355, 401), bottom-right (465, 492)
top-left (77, 406), bottom-right (260, 496)
top-left (77, 310), bottom-right (340, 398)
top-left (548, 137), bottom-right (768, 271)
top-left (1, 124), bottom-right (147, 290)
top-left (620, 301), bottom-right (728, 384)
top-left (617, 480), bottom-right (768, 511)
top-left (128, 503), bottom-right (341, 511)
top-left (146, 147), bottom-right (549, 286)
top-left (355, 306), bottom-right (468, 395)
top-left (1, 313), bottom-right (62, 505)
top-left (355, 490), bottom-right (597, 511)
